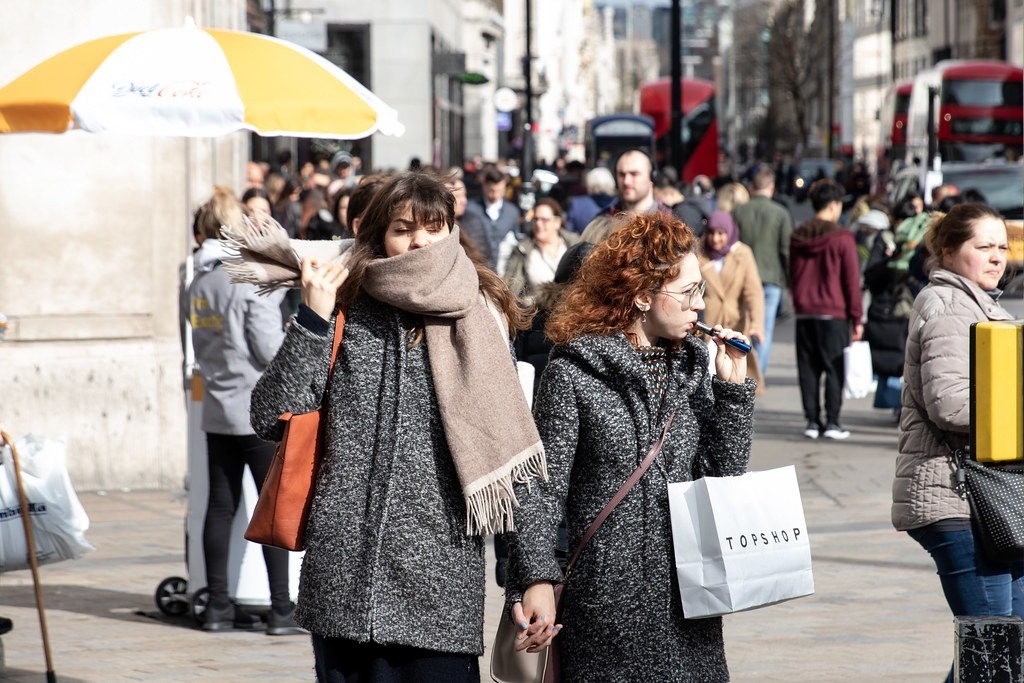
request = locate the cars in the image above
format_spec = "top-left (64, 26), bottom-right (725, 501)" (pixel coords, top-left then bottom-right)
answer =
top-left (794, 158), bottom-right (852, 202)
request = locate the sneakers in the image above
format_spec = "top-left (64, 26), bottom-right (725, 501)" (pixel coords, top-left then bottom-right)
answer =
top-left (266, 607), bottom-right (312, 635)
top-left (203, 603), bottom-right (261, 630)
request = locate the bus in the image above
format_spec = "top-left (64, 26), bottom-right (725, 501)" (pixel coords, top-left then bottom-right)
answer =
top-left (632, 78), bottom-right (721, 184)
top-left (876, 58), bottom-right (1023, 268)
top-left (583, 112), bottom-right (656, 176)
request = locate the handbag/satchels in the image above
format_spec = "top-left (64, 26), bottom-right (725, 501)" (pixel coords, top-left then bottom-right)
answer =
top-left (667, 465), bottom-right (815, 619)
top-left (490, 583), bottom-right (564, 683)
top-left (244, 303), bottom-right (345, 551)
top-left (953, 457), bottom-right (1024, 571)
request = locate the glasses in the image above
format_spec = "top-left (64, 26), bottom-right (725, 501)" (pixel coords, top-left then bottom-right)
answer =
top-left (638, 280), bottom-right (707, 308)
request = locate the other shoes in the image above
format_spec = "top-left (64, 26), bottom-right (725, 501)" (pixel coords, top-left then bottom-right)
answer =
top-left (823, 423), bottom-right (850, 439)
top-left (805, 421), bottom-right (824, 438)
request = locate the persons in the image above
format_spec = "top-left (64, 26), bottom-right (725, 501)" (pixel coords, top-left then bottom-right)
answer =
top-left (250, 174), bottom-right (563, 683)
top-left (495, 211), bottom-right (756, 683)
top-left (0, 431), bottom-right (16, 635)
top-left (891, 201), bottom-right (1024, 683)
top-left (177, 145), bottom-right (957, 576)
top-left (189, 189), bottom-right (323, 636)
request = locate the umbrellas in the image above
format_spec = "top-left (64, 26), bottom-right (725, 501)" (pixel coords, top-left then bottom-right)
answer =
top-left (0, 16), bottom-right (409, 141)
